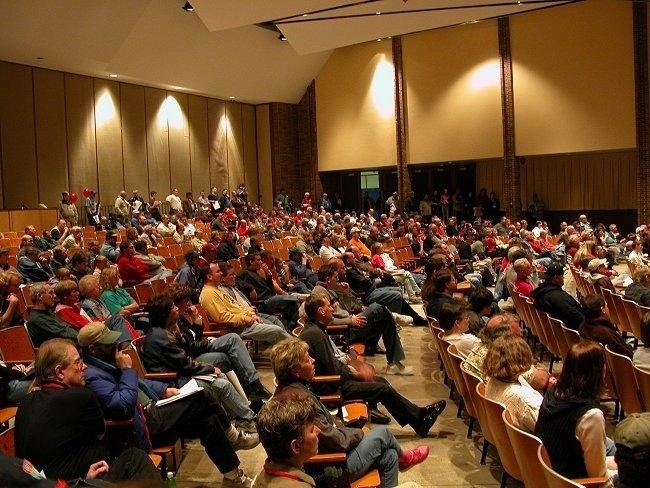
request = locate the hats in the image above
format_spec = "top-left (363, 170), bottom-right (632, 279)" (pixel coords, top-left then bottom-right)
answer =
top-left (184, 250), bottom-right (201, 263)
top-left (614, 410), bottom-right (650, 450)
top-left (290, 247), bottom-right (305, 252)
top-left (539, 264), bottom-right (563, 280)
top-left (349, 226), bottom-right (361, 234)
top-left (244, 237), bottom-right (257, 249)
top-left (587, 257), bottom-right (607, 274)
top-left (77, 321), bottom-right (122, 347)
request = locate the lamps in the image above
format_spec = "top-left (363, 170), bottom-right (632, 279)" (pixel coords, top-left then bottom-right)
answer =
top-left (277, 32), bottom-right (287, 40)
top-left (181, 1), bottom-right (193, 12)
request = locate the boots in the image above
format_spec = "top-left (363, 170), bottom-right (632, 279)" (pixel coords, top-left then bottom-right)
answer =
top-left (251, 378), bottom-right (272, 398)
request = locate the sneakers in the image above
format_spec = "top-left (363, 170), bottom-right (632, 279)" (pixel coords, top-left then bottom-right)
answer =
top-left (393, 312), bottom-right (413, 326)
top-left (375, 345), bottom-right (387, 354)
top-left (222, 469), bottom-right (252, 488)
top-left (235, 418), bottom-right (258, 433)
top-left (400, 444), bottom-right (429, 472)
top-left (386, 362), bottom-right (414, 376)
top-left (233, 430), bottom-right (261, 451)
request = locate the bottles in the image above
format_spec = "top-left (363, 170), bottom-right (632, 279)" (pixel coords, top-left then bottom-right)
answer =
top-left (165, 472), bottom-right (178, 488)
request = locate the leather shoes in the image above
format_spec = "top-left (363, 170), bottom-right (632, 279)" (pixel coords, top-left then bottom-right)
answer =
top-left (420, 408), bottom-right (440, 437)
top-left (371, 408), bottom-right (391, 424)
top-left (425, 400), bottom-right (446, 415)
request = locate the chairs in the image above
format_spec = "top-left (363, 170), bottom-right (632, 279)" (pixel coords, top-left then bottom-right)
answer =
top-left (0, 219), bottom-right (649, 488)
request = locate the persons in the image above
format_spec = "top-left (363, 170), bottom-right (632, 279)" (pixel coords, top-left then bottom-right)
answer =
top-left (1, 177), bottom-right (648, 484)
